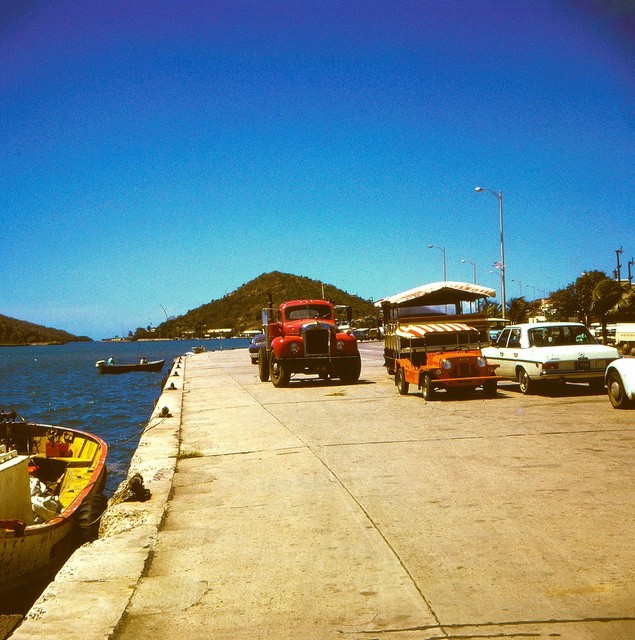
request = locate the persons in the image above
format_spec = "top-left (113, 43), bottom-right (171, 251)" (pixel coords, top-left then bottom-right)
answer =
top-left (140, 357), bottom-right (147, 365)
top-left (107, 356), bottom-right (115, 365)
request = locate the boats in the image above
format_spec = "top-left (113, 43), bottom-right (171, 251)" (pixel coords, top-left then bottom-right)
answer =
top-left (95, 358), bottom-right (166, 374)
top-left (0, 407), bottom-right (109, 596)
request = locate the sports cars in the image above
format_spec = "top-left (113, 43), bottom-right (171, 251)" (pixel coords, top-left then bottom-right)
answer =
top-left (604, 358), bottom-right (635, 408)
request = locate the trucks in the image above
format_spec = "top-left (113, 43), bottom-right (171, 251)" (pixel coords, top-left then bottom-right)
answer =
top-left (258, 299), bottom-right (361, 388)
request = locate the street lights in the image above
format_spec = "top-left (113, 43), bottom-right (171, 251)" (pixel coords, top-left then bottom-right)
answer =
top-left (512, 280), bottom-right (522, 297)
top-left (461, 259), bottom-right (476, 284)
top-left (526, 284), bottom-right (546, 300)
top-left (490, 271), bottom-right (501, 276)
top-left (428, 245), bottom-right (447, 314)
top-left (474, 186), bottom-right (507, 328)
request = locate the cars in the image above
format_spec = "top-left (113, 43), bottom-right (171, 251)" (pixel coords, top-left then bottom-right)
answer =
top-left (248, 334), bottom-right (266, 365)
top-left (480, 321), bottom-right (621, 395)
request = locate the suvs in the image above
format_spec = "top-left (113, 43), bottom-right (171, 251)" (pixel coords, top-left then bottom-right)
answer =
top-left (393, 322), bottom-right (503, 401)
